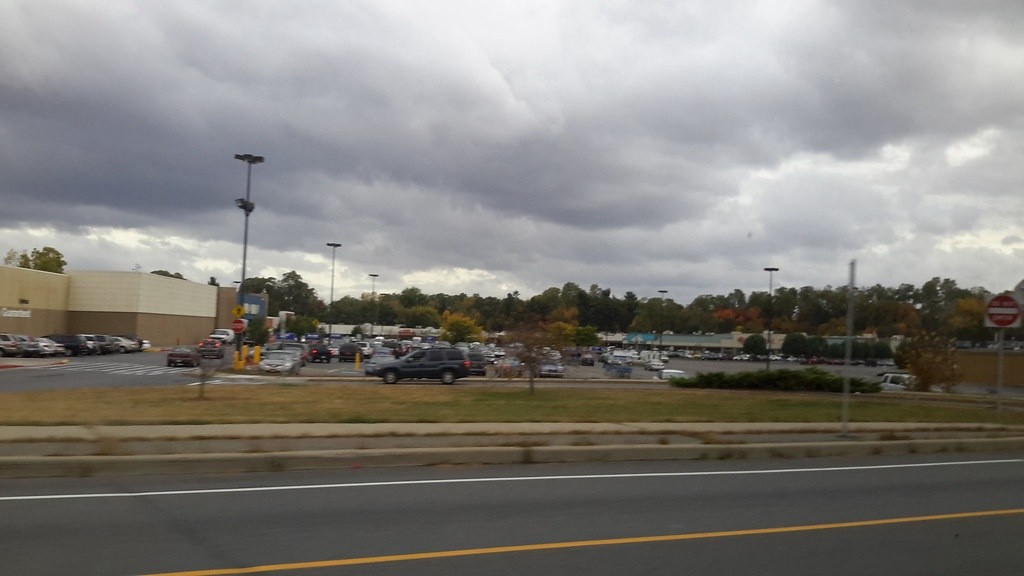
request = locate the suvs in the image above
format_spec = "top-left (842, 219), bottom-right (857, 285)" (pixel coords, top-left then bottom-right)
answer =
top-left (374, 345), bottom-right (471, 384)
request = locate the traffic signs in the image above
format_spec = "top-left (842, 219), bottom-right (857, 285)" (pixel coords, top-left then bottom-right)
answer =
top-left (231, 319), bottom-right (245, 333)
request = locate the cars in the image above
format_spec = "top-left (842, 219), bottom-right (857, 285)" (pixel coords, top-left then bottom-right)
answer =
top-left (258, 331), bottom-right (944, 395)
top-left (167, 345), bottom-right (202, 367)
top-left (0, 332), bottom-right (150, 358)
top-left (198, 338), bottom-right (225, 359)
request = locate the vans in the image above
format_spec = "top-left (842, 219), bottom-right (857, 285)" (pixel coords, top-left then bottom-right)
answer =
top-left (209, 329), bottom-right (235, 345)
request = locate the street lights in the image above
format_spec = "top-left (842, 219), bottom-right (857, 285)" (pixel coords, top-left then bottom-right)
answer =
top-left (764, 268), bottom-right (779, 354)
top-left (326, 243), bottom-right (341, 345)
top-left (369, 274), bottom-right (379, 339)
top-left (658, 290), bottom-right (668, 359)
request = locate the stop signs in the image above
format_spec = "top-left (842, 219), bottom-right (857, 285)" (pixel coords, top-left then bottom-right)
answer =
top-left (983, 293), bottom-right (1023, 327)
